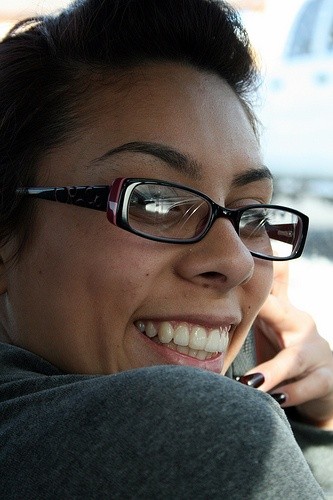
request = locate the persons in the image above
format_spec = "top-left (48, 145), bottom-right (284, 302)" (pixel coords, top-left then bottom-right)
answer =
top-left (0, 0), bottom-right (333, 500)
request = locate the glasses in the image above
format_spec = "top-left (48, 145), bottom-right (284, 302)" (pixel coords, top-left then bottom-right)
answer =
top-left (16, 176), bottom-right (309, 261)
